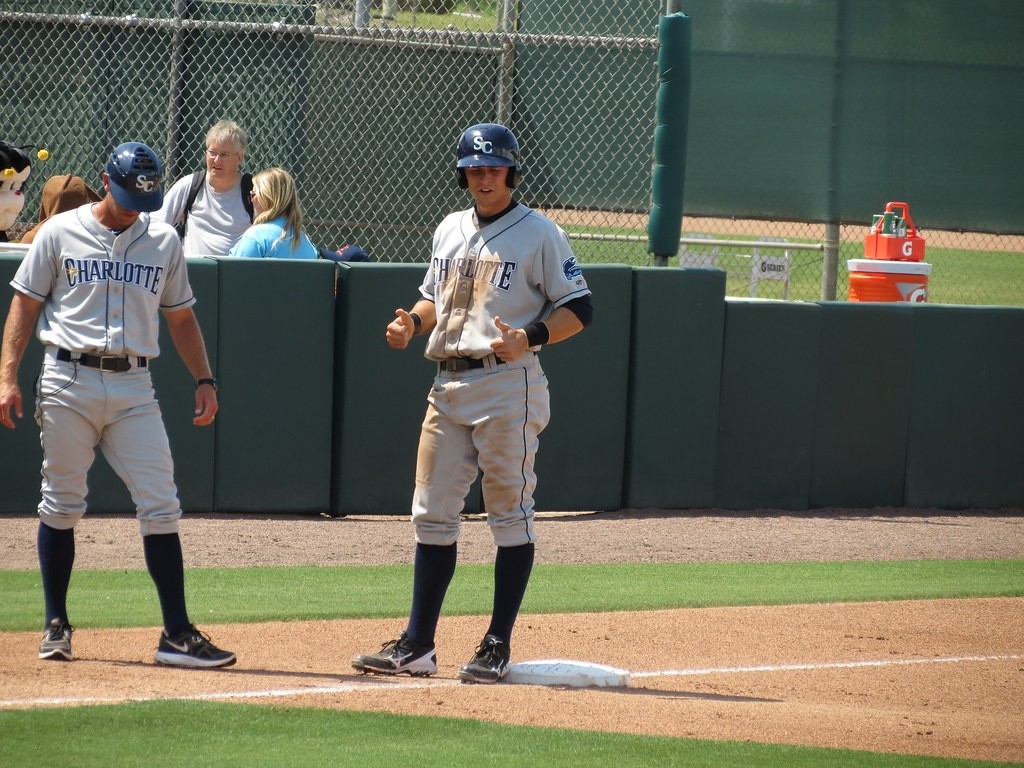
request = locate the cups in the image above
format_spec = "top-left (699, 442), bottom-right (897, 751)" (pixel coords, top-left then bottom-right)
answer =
top-left (871, 215), bottom-right (883, 234)
top-left (884, 212), bottom-right (894, 234)
top-left (895, 219), bottom-right (906, 238)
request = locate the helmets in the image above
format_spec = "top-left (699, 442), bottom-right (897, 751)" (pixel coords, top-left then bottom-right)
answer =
top-left (106, 142), bottom-right (165, 212)
top-left (455, 123), bottom-right (522, 188)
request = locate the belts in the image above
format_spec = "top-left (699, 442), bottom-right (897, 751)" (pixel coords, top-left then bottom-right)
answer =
top-left (440, 353), bottom-right (507, 374)
top-left (57, 348), bottom-right (147, 372)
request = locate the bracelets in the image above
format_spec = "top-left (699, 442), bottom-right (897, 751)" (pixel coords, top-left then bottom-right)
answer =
top-left (196, 377), bottom-right (218, 390)
top-left (522, 322), bottom-right (551, 352)
top-left (409, 312), bottom-right (425, 336)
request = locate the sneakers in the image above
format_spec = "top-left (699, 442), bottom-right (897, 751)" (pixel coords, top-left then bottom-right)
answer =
top-left (459, 634), bottom-right (510, 684)
top-left (351, 633), bottom-right (437, 676)
top-left (154, 624), bottom-right (237, 668)
top-left (39, 615), bottom-right (75, 660)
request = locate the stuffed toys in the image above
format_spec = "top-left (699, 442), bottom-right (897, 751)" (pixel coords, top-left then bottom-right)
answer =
top-left (2, 131), bottom-right (49, 243)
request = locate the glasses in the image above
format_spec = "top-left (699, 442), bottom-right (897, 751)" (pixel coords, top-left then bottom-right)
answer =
top-left (205, 148), bottom-right (237, 161)
top-left (250, 191), bottom-right (257, 200)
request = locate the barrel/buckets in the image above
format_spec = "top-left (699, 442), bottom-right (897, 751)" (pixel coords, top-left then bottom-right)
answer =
top-left (847, 260), bottom-right (932, 303)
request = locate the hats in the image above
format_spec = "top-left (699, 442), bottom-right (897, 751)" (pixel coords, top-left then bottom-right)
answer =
top-left (319, 244), bottom-right (370, 262)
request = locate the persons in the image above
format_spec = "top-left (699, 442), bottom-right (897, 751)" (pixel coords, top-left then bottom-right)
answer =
top-left (20, 118), bottom-right (371, 261)
top-left (350, 123), bottom-right (594, 685)
top-left (1, 140), bottom-right (236, 668)
